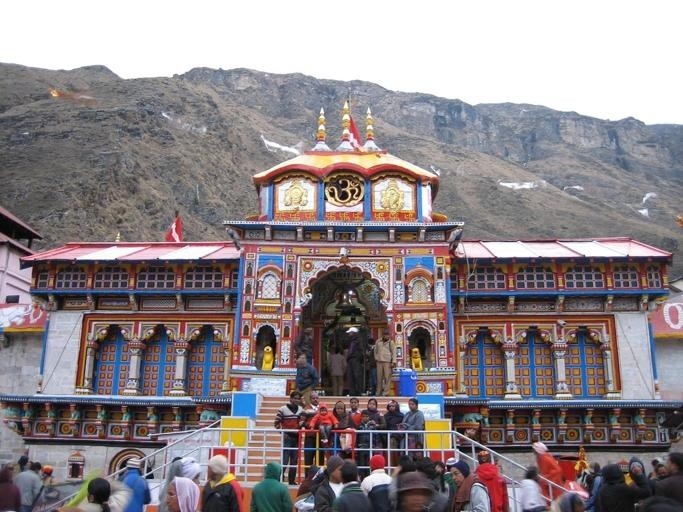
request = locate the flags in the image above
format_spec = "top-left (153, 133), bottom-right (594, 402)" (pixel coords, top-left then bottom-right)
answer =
top-left (164, 215), bottom-right (181, 243)
top-left (349, 115), bottom-right (361, 153)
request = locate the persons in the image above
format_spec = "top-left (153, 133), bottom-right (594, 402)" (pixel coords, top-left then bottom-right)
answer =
top-left (290, 322), bottom-right (397, 397)
top-left (383, 399), bottom-right (404, 466)
top-left (292, 353), bottom-right (319, 406)
top-left (357, 409), bottom-right (377, 475)
top-left (0, 439), bottom-right (682, 511)
top-left (328, 400), bottom-right (349, 457)
top-left (302, 391), bottom-right (325, 480)
top-left (308, 404), bottom-right (339, 443)
top-left (347, 397), bottom-right (361, 428)
top-left (362, 397), bottom-right (386, 458)
top-left (399, 397), bottom-right (425, 458)
top-left (273, 390), bottom-right (307, 486)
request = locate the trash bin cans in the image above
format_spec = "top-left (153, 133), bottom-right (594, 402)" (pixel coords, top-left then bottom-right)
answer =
top-left (399, 371), bottom-right (417, 397)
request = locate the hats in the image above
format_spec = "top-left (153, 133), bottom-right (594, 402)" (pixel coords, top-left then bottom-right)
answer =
top-left (326, 450), bottom-right (472, 500)
top-left (532, 441), bottom-right (549, 454)
top-left (126, 458), bottom-right (140, 469)
top-left (44, 465), bottom-right (53, 473)
top-left (208, 455), bottom-right (228, 475)
